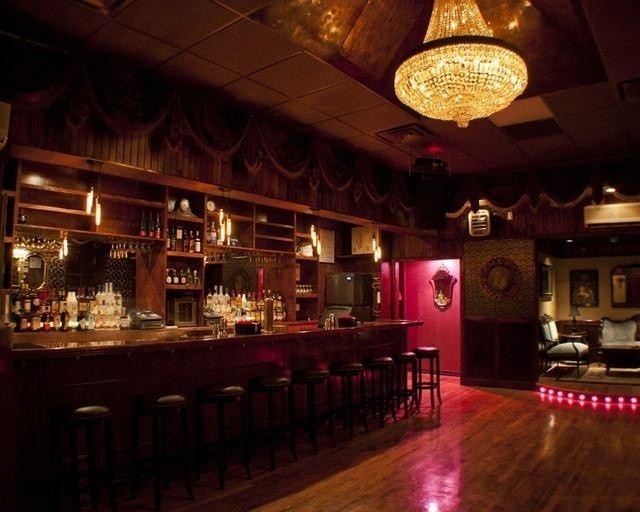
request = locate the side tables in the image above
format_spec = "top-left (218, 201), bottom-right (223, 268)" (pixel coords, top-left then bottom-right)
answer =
top-left (558, 330), bottom-right (590, 343)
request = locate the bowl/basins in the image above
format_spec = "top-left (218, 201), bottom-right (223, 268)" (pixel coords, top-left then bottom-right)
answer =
top-left (167, 199), bottom-right (176, 212)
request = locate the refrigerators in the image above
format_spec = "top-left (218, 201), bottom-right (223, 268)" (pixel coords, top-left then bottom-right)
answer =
top-left (325, 271), bottom-right (373, 322)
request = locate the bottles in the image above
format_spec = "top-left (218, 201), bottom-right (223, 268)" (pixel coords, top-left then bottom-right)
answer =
top-left (147, 210), bottom-right (155, 238)
top-left (9, 282), bottom-right (130, 330)
top-left (164, 267), bottom-right (202, 287)
top-left (155, 212), bottom-right (162, 238)
top-left (110, 241), bottom-right (127, 258)
top-left (139, 209), bottom-right (147, 236)
top-left (206, 281), bottom-right (315, 335)
top-left (206, 218), bottom-right (217, 244)
top-left (166, 226), bottom-right (203, 253)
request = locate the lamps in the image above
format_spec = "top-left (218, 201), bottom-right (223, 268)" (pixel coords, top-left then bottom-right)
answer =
top-left (372, 223), bottom-right (382, 263)
top-left (569, 305), bottom-right (581, 334)
top-left (394, 0), bottom-right (529, 128)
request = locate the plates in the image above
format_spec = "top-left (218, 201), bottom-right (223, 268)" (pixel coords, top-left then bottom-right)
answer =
top-left (207, 200), bottom-right (216, 211)
top-left (180, 198), bottom-right (190, 212)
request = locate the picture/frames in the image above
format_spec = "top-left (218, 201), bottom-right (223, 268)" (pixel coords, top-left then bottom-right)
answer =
top-left (539, 262), bottom-right (554, 296)
top-left (569, 268), bottom-right (599, 308)
top-left (610, 263), bottom-right (640, 308)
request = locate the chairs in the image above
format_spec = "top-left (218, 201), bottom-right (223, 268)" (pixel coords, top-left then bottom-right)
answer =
top-left (598, 316), bottom-right (638, 368)
top-left (540, 314), bottom-right (590, 382)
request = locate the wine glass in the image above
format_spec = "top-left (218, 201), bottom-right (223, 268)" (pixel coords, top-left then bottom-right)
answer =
top-left (204, 246), bottom-right (283, 270)
top-left (129, 239), bottom-right (155, 257)
